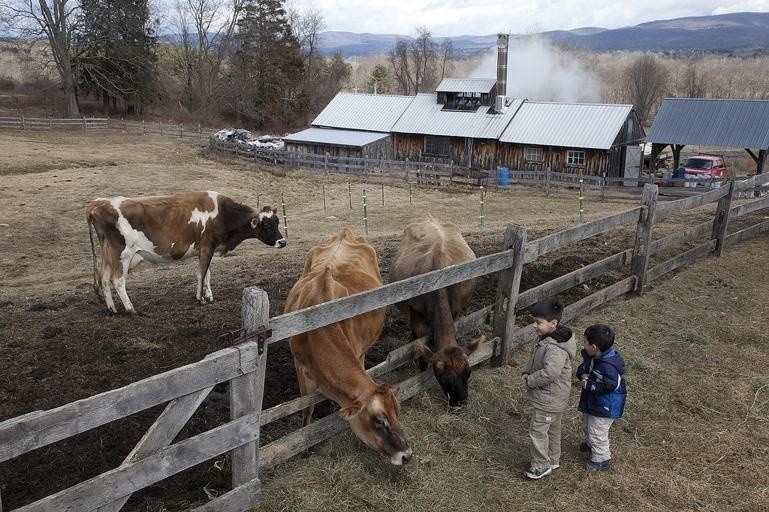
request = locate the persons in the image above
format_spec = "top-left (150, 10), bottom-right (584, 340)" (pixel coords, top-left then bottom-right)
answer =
top-left (655, 158), bottom-right (687, 188)
top-left (576, 324), bottom-right (627, 473)
top-left (519, 298), bottom-right (577, 479)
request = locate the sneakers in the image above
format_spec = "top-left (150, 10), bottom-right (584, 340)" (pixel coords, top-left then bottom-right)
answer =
top-left (584, 459), bottom-right (611, 471)
top-left (580, 442), bottom-right (592, 452)
top-left (525, 460), bottom-right (559, 479)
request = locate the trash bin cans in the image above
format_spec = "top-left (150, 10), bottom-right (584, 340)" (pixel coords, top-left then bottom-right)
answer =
top-left (497, 166), bottom-right (510, 186)
top-left (672, 168), bottom-right (685, 186)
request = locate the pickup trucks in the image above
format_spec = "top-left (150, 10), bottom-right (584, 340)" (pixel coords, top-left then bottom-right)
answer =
top-left (677, 156), bottom-right (735, 184)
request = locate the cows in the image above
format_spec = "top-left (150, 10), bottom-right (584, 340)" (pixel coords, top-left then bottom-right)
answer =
top-left (282, 225), bottom-right (414, 468)
top-left (86, 189), bottom-right (288, 318)
top-left (387, 211), bottom-right (487, 416)
